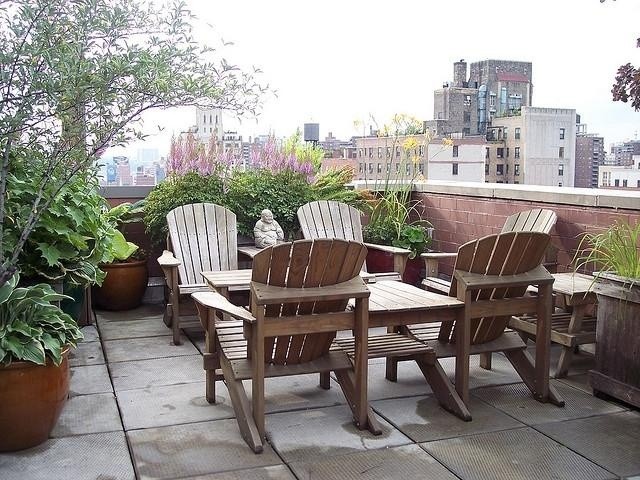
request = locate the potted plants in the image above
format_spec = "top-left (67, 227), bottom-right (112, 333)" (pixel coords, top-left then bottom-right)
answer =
top-left (386, 222), bottom-right (433, 284)
top-left (0, 271), bottom-right (85, 452)
top-left (93, 199), bottom-right (152, 311)
top-left (569, 216), bottom-right (640, 409)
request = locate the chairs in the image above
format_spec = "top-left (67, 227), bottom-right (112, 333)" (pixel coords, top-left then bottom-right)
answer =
top-left (420, 207), bottom-right (558, 296)
top-left (387, 230), bottom-right (565, 411)
top-left (297, 200), bottom-right (410, 280)
top-left (156, 202), bottom-right (238, 345)
top-left (191, 238), bottom-right (382, 454)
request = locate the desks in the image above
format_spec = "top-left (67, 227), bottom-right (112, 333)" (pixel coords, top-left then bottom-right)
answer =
top-left (238, 245), bottom-right (294, 259)
top-left (199, 268), bottom-right (376, 321)
top-left (522, 271), bottom-right (600, 378)
top-left (333, 280), bottom-right (465, 361)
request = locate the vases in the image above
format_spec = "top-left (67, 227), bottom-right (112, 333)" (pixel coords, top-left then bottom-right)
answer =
top-left (366, 246), bottom-right (386, 273)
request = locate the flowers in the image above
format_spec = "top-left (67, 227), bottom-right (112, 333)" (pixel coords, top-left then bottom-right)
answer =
top-left (353, 112), bottom-right (454, 245)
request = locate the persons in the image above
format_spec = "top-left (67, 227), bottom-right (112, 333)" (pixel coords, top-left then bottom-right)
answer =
top-left (252, 209), bottom-right (284, 249)
